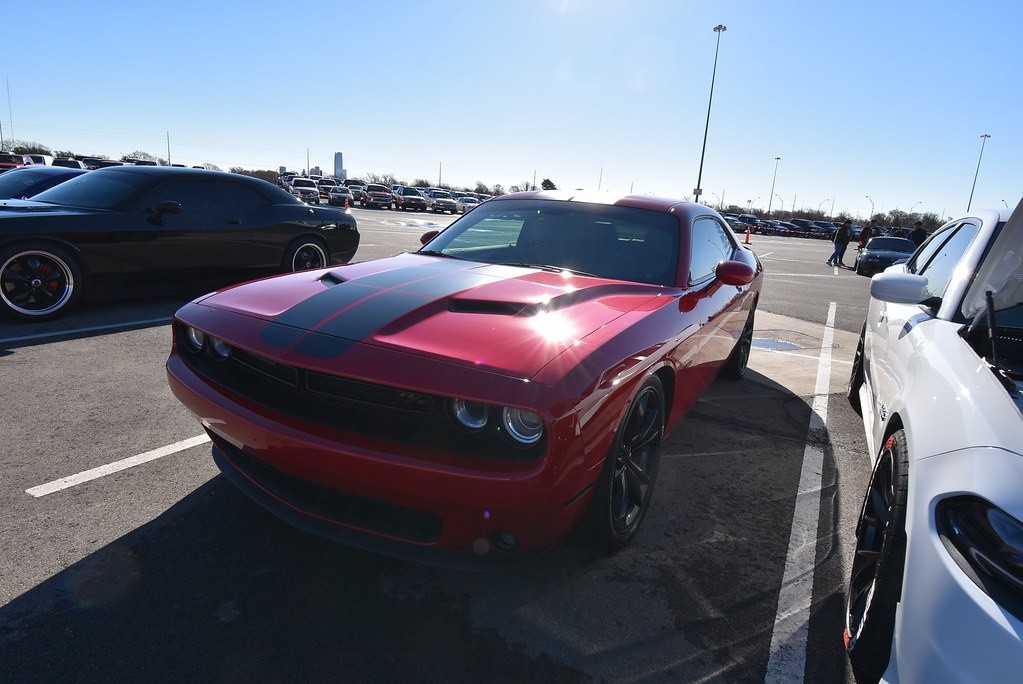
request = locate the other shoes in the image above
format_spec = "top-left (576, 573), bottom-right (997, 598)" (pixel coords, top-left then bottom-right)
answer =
top-left (834, 263), bottom-right (841, 267)
top-left (826, 260), bottom-right (832, 266)
top-left (839, 263), bottom-right (845, 266)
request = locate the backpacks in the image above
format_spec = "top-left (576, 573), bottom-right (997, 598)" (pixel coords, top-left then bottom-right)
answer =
top-left (830, 227), bottom-right (842, 242)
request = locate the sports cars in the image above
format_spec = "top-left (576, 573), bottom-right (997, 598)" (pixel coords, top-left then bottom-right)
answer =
top-left (166, 190), bottom-right (764, 572)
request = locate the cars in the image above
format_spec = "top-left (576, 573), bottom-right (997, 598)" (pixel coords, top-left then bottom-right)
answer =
top-left (275, 171), bottom-right (494, 214)
top-left (847, 195), bottom-right (1023, 683)
top-left (0, 151), bottom-right (204, 200)
top-left (719, 212), bottom-right (934, 240)
top-left (854, 236), bottom-right (917, 275)
top-left (0, 166), bottom-right (360, 323)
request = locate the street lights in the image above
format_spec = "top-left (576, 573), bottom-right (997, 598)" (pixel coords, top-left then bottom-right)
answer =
top-left (768, 156), bottom-right (781, 213)
top-left (967, 133), bottom-right (990, 213)
top-left (819, 199), bottom-right (830, 210)
top-left (775, 194), bottom-right (783, 211)
top-left (910, 202), bottom-right (922, 216)
top-left (866, 195), bottom-right (874, 220)
top-left (695, 24), bottom-right (728, 204)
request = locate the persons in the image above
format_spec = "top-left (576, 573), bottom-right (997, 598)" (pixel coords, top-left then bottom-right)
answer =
top-left (859, 220), bottom-right (880, 253)
top-left (826, 219), bottom-right (852, 266)
top-left (906, 220), bottom-right (926, 248)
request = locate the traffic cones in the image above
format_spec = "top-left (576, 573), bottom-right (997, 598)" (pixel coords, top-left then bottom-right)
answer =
top-left (742, 231), bottom-right (752, 245)
